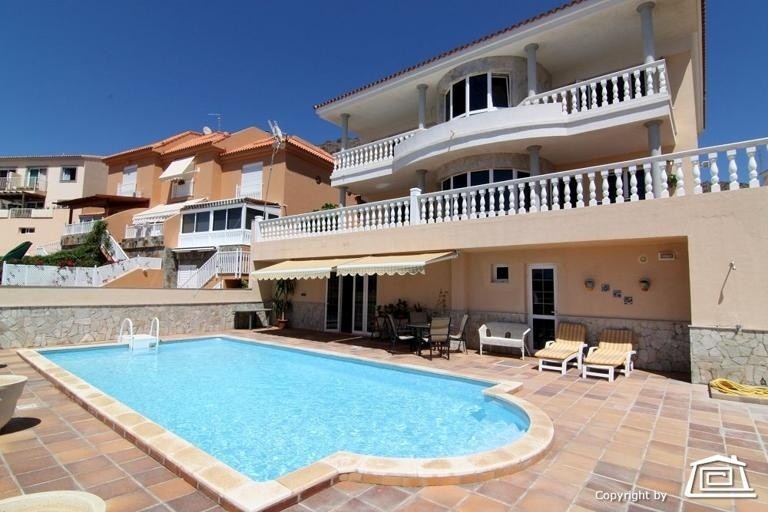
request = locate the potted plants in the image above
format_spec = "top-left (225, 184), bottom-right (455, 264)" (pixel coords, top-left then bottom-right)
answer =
top-left (396, 298), bottom-right (409, 319)
top-left (272, 278), bottom-right (295, 328)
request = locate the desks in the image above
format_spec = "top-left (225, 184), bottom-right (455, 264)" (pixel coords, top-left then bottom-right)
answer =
top-left (236, 308), bottom-right (273, 330)
top-left (371, 315), bottom-right (408, 343)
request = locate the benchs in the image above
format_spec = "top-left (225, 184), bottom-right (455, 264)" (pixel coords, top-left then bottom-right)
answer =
top-left (478, 321), bottom-right (531, 359)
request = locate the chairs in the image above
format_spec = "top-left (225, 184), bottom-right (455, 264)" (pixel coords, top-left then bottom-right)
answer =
top-left (535, 322), bottom-right (588, 375)
top-left (385, 312), bottom-right (469, 361)
top-left (582, 329), bottom-right (637, 383)
